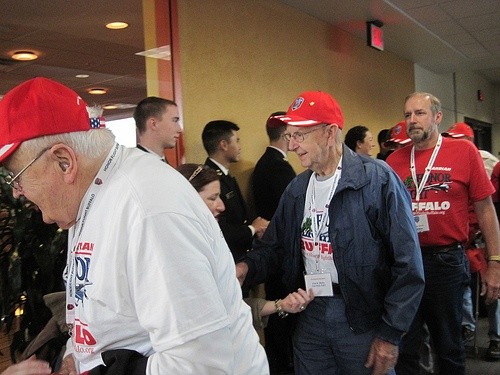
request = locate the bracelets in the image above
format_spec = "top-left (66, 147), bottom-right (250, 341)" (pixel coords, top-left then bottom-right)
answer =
top-left (275, 298), bottom-right (288, 318)
top-left (488, 256), bottom-right (500, 261)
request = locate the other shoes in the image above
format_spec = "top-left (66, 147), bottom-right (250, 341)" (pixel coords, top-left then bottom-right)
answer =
top-left (462, 328), bottom-right (474, 343)
top-left (487, 342), bottom-right (500, 363)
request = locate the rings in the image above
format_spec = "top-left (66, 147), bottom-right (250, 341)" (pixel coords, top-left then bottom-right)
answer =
top-left (300, 306), bottom-right (305, 310)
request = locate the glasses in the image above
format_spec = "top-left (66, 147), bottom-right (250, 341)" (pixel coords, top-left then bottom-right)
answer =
top-left (4, 146), bottom-right (52, 192)
top-left (282, 125), bottom-right (330, 144)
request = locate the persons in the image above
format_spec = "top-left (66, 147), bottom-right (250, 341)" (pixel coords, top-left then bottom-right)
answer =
top-left (440, 119), bottom-right (500, 355)
top-left (175, 164), bottom-right (314, 326)
top-left (376, 120), bottom-right (412, 162)
top-left (134, 96), bottom-right (182, 165)
top-left (0, 201), bottom-right (68, 375)
top-left (345, 126), bottom-right (375, 157)
top-left (386, 92), bottom-right (500, 375)
top-left (0, 77), bottom-right (271, 375)
top-left (234, 90), bottom-right (426, 375)
top-left (201, 120), bottom-right (270, 299)
top-left (249, 111), bottom-right (297, 302)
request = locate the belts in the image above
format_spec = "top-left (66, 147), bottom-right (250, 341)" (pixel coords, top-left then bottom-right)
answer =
top-left (421, 243), bottom-right (462, 254)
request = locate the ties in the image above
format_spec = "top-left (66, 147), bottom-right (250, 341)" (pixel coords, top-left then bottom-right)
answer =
top-left (227, 173), bottom-right (234, 184)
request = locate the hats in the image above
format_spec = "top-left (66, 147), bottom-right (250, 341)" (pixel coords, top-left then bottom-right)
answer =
top-left (0, 77), bottom-right (106, 164)
top-left (441, 123), bottom-right (474, 140)
top-left (384, 122), bottom-right (414, 149)
top-left (267, 91), bottom-right (344, 131)
